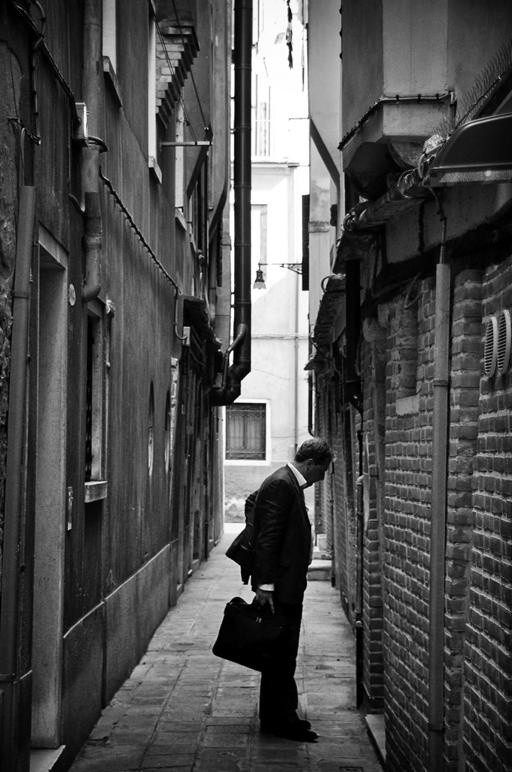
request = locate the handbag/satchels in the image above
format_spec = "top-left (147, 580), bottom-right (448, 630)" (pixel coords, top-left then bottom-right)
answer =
top-left (211, 596), bottom-right (282, 673)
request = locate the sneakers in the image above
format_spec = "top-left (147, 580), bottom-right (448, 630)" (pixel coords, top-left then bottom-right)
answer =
top-left (259, 719), bottom-right (318, 741)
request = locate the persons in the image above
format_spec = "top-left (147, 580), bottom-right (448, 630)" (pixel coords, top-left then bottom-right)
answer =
top-left (250, 437), bottom-right (335, 742)
